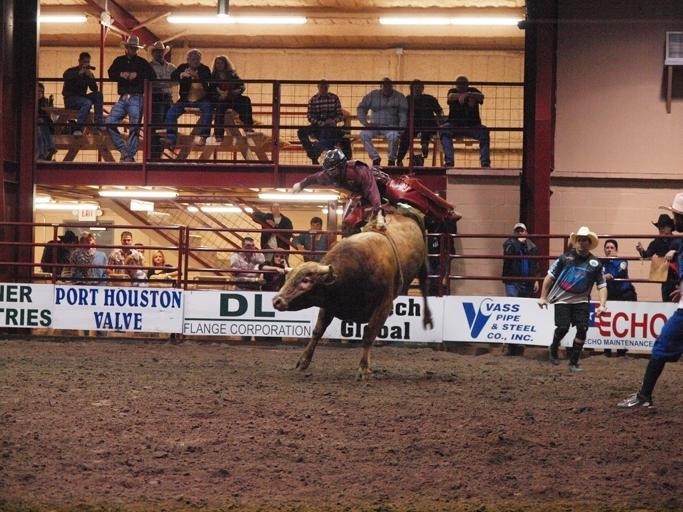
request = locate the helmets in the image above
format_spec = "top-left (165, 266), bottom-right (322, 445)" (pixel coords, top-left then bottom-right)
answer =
top-left (322, 149), bottom-right (347, 172)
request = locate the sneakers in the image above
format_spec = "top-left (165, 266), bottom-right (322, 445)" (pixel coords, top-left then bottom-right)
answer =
top-left (72, 126), bottom-right (105, 137)
top-left (503, 346), bottom-right (524, 356)
top-left (118, 154), bottom-right (134, 162)
top-left (158, 127), bottom-right (255, 151)
top-left (311, 158), bottom-right (493, 167)
top-left (615, 354), bottom-right (635, 360)
top-left (616, 392), bottom-right (657, 408)
top-left (437, 209), bottom-right (462, 222)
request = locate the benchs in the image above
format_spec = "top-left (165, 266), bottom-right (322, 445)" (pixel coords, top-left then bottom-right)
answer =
top-left (44, 108), bottom-right (114, 162)
top-left (346, 114), bottom-right (479, 168)
top-left (175, 108), bottom-right (268, 161)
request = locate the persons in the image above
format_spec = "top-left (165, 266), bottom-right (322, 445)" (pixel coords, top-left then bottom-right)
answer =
top-left (636, 214), bottom-right (680, 301)
top-left (601, 240), bottom-right (636, 358)
top-left (502, 224), bottom-right (539, 355)
top-left (537, 227), bottom-right (606, 370)
top-left (295, 76), bottom-right (490, 170)
top-left (36, 36), bottom-right (262, 162)
top-left (616, 192), bottom-right (683, 408)
top-left (41, 201), bottom-right (458, 342)
top-left (293, 149), bottom-right (463, 220)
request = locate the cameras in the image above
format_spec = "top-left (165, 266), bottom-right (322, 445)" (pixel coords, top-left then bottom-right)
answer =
top-left (86, 65), bottom-right (95, 70)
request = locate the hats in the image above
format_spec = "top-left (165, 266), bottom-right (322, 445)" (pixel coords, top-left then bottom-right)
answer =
top-left (514, 223), bottom-right (526, 231)
top-left (57, 230), bottom-right (94, 240)
top-left (651, 214), bottom-right (675, 232)
top-left (569, 227), bottom-right (599, 250)
top-left (150, 41), bottom-right (171, 57)
top-left (658, 193), bottom-right (683, 215)
top-left (121, 35), bottom-right (146, 49)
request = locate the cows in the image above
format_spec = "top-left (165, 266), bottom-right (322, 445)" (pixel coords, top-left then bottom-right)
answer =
top-left (272, 201), bottom-right (434, 382)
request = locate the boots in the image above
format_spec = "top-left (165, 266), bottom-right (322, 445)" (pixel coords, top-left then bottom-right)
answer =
top-left (567, 340), bottom-right (584, 371)
top-left (549, 334), bottom-right (561, 365)
